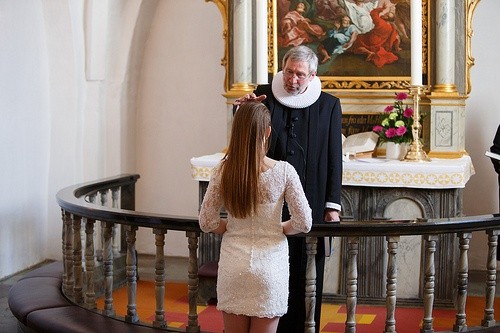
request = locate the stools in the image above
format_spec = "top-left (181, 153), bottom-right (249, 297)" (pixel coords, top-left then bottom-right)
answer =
top-left (197, 262), bottom-right (219, 305)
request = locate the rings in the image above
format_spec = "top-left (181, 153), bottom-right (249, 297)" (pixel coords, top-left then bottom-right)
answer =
top-left (234, 99), bottom-right (240, 103)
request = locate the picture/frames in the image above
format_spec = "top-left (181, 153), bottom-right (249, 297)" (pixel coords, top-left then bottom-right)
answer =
top-left (267, 0), bottom-right (432, 98)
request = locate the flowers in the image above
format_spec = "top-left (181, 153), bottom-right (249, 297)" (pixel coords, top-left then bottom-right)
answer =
top-left (373, 92), bottom-right (428, 143)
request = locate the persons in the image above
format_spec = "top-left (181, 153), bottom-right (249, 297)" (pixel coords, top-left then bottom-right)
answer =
top-left (232, 46), bottom-right (342, 333)
top-left (198, 101), bottom-right (312, 333)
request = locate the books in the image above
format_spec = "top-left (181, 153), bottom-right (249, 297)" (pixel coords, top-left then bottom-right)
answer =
top-left (341, 131), bottom-right (380, 155)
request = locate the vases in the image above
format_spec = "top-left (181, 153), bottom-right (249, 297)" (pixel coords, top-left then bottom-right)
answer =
top-left (386, 142), bottom-right (408, 160)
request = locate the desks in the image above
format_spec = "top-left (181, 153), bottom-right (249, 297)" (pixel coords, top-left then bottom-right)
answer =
top-left (190, 152), bottom-right (476, 309)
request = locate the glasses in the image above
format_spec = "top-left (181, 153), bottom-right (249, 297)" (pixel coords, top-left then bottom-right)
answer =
top-left (282, 68), bottom-right (310, 80)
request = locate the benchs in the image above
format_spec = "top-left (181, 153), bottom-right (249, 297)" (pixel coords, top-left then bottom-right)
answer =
top-left (7, 260), bottom-right (184, 333)
